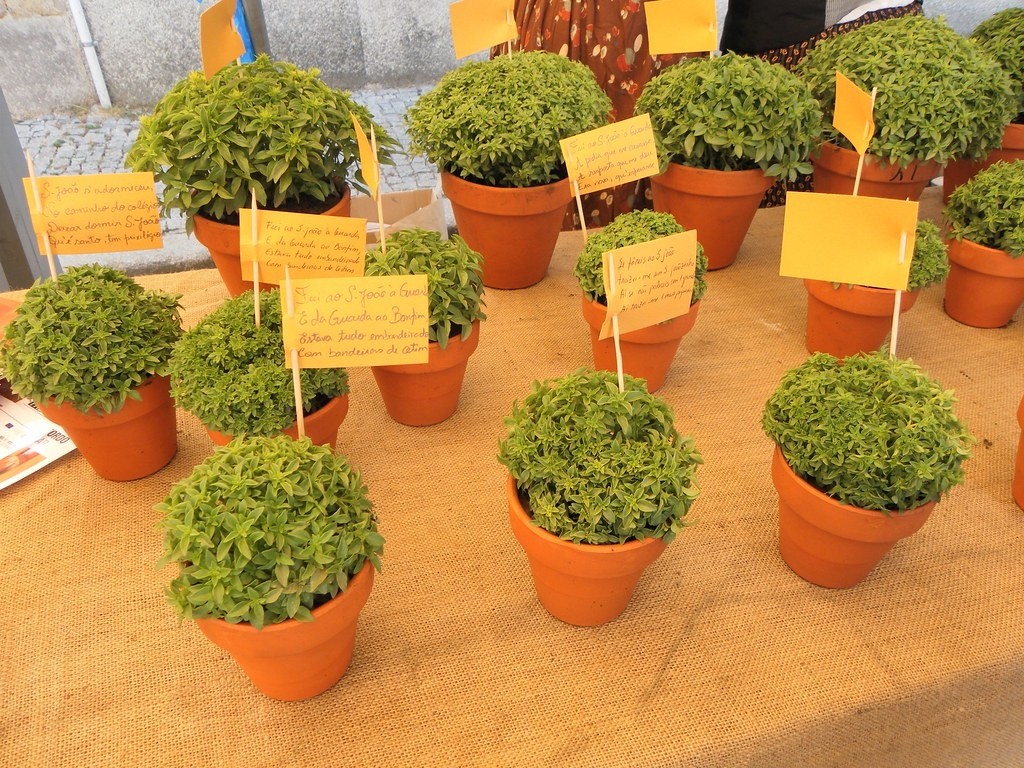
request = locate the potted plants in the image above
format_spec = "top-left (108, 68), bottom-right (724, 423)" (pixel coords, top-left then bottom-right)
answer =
top-left (573, 210), bottom-right (707, 394)
top-left (796, 17), bottom-right (1015, 196)
top-left (168, 289), bottom-right (348, 461)
top-left (763, 353), bottom-right (974, 590)
top-left (407, 51), bottom-right (616, 290)
top-left (804, 216), bottom-right (952, 364)
top-left (0, 268), bottom-right (184, 483)
top-left (942, 159), bottom-right (1024, 332)
top-left (636, 51), bottom-right (820, 271)
top-left (153, 434), bottom-right (382, 702)
top-left (969, 0), bottom-right (1024, 176)
top-left (368, 230), bottom-right (488, 427)
top-left (123, 56), bottom-right (406, 301)
top-left (495, 370), bottom-right (699, 629)
